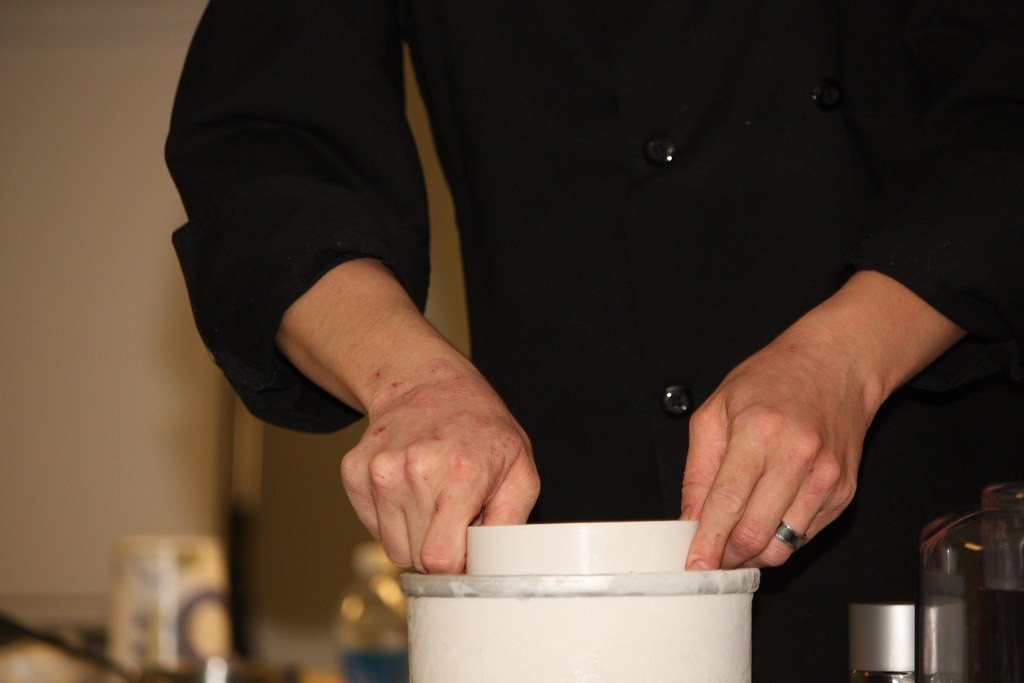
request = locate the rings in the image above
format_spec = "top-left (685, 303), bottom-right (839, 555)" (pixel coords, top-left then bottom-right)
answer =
top-left (776, 522), bottom-right (807, 550)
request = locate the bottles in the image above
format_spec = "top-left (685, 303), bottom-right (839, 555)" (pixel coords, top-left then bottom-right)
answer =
top-left (919, 479), bottom-right (1024, 683)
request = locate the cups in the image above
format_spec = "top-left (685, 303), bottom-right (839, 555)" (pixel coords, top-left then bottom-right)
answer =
top-left (398, 568), bottom-right (762, 683)
top-left (465, 519), bottom-right (700, 576)
top-left (105, 534), bottom-right (229, 676)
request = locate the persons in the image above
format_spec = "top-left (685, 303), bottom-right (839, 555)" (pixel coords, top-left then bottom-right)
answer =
top-left (164, 0), bottom-right (1024, 683)
